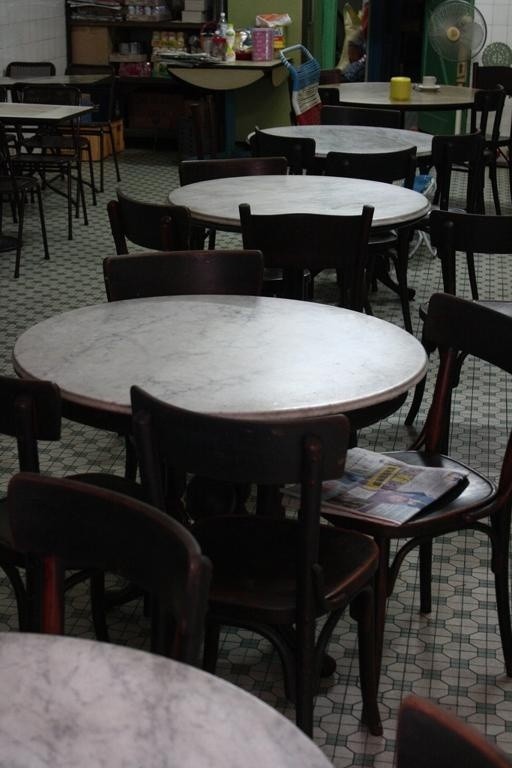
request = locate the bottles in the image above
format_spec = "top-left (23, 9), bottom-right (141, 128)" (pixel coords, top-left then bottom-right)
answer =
top-left (115, 0), bottom-right (253, 79)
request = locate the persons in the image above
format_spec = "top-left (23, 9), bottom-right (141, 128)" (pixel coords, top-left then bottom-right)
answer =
top-left (386, 493), bottom-right (436, 509)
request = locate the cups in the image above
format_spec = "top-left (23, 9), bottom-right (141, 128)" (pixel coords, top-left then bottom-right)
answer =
top-left (389, 77), bottom-right (411, 101)
top-left (422, 76), bottom-right (437, 87)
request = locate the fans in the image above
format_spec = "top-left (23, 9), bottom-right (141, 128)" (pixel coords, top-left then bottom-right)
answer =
top-left (425, 0), bottom-right (491, 84)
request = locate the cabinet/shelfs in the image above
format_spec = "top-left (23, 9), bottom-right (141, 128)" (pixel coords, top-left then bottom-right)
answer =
top-left (61, 0), bottom-right (233, 158)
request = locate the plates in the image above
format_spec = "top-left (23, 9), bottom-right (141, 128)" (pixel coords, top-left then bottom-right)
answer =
top-left (416, 85), bottom-right (441, 91)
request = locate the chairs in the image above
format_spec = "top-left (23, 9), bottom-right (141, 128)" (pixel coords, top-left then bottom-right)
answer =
top-left (4, 52), bottom-right (511, 425)
top-left (0, 293), bottom-right (512, 768)
